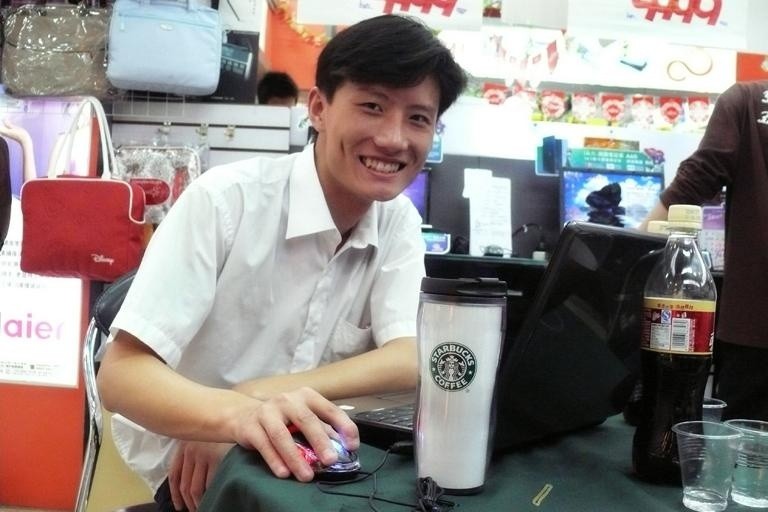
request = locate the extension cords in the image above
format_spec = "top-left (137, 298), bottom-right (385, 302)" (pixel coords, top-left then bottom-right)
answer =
top-left (533, 251), bottom-right (551, 261)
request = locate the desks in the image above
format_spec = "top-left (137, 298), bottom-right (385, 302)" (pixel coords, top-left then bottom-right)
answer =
top-left (227, 411), bottom-right (768, 512)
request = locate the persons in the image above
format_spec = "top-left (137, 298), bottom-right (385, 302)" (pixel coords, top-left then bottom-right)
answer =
top-left (94, 14), bottom-right (470, 510)
top-left (632, 81), bottom-right (767, 430)
top-left (259, 69), bottom-right (301, 109)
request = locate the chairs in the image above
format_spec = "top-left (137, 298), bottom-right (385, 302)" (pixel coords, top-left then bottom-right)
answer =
top-left (57, 268), bottom-right (158, 512)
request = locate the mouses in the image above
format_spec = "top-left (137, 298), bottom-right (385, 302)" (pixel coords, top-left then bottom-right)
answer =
top-left (290, 431), bottom-right (362, 482)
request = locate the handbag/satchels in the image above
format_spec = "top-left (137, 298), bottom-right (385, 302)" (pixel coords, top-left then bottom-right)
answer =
top-left (106, 0), bottom-right (223, 97)
top-left (18, 94), bottom-right (148, 283)
top-left (1, 0), bottom-right (122, 101)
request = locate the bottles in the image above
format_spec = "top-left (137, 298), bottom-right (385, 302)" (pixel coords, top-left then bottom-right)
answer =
top-left (629, 205), bottom-right (717, 489)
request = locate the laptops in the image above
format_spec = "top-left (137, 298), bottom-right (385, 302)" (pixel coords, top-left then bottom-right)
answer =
top-left (320, 221), bottom-right (670, 461)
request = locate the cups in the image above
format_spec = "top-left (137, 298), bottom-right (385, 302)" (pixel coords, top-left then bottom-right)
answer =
top-left (411, 276), bottom-right (510, 494)
top-left (674, 418), bottom-right (768, 511)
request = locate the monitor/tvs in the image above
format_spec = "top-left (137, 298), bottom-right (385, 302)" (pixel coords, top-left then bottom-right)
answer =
top-left (560, 168), bottom-right (665, 234)
top-left (404, 167), bottom-right (432, 223)
